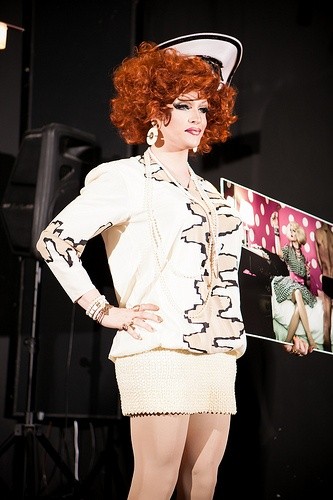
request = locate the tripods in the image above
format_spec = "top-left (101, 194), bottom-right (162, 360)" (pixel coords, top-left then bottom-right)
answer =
top-left (0, 424), bottom-right (84, 500)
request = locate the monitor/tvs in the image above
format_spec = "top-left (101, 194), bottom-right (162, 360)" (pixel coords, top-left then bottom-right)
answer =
top-left (217, 177), bottom-right (333, 357)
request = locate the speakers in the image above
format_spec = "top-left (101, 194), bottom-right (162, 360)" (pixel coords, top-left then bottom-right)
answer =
top-left (9, 256), bottom-right (124, 420)
top-left (0, 124), bottom-right (106, 259)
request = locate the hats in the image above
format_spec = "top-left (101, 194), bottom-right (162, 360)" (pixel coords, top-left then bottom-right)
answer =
top-left (148, 31), bottom-right (243, 91)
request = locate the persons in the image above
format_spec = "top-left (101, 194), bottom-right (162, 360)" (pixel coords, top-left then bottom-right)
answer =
top-left (35, 40), bottom-right (247, 500)
top-left (314, 223), bottom-right (333, 352)
top-left (268, 212), bottom-right (318, 353)
